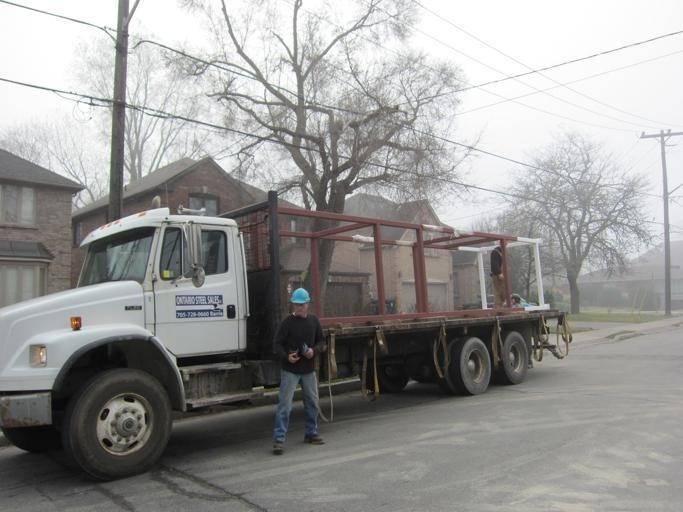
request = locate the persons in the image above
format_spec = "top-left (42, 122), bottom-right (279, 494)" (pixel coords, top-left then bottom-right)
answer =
top-left (271, 288), bottom-right (324, 453)
top-left (490, 245), bottom-right (504, 307)
top-left (510, 293), bottom-right (531, 311)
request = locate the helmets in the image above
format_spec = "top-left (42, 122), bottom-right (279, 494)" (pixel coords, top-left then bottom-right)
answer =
top-left (290, 288), bottom-right (310, 304)
top-left (511, 294), bottom-right (520, 303)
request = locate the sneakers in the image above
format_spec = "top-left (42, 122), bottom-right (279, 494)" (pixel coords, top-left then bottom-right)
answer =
top-left (272, 442), bottom-right (284, 452)
top-left (304, 434), bottom-right (325, 444)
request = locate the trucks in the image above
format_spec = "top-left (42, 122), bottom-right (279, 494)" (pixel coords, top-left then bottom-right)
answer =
top-left (0, 193), bottom-right (576, 480)
